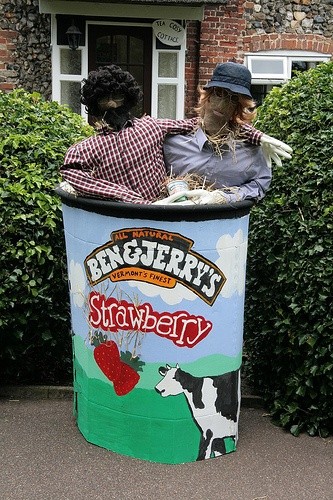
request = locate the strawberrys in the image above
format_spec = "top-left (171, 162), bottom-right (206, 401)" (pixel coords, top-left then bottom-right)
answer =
top-left (113, 351), bottom-right (145, 396)
top-left (91, 332), bottom-right (120, 381)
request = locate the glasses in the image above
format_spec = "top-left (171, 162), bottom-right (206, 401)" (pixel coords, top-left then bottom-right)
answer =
top-left (212, 87), bottom-right (244, 105)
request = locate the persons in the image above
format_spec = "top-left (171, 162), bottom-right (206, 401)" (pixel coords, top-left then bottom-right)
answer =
top-left (59, 62), bottom-right (294, 205)
top-left (163, 62), bottom-right (272, 205)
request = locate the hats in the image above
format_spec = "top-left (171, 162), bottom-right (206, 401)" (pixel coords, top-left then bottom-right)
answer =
top-left (202, 62), bottom-right (254, 99)
